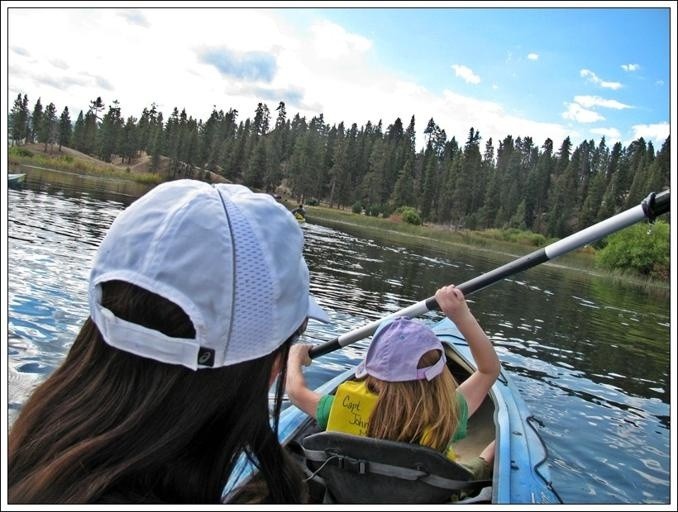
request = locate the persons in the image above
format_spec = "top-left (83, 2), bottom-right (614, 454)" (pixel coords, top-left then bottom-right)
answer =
top-left (285, 285), bottom-right (501, 502)
top-left (8, 179), bottom-right (312, 503)
top-left (291, 204), bottom-right (307, 219)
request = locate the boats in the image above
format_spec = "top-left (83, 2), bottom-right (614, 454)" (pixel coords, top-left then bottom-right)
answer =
top-left (221, 315), bottom-right (558, 503)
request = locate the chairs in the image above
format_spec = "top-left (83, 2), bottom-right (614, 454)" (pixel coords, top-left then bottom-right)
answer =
top-left (299, 428), bottom-right (475, 505)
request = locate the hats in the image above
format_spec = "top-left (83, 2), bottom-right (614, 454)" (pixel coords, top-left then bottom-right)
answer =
top-left (88, 178), bottom-right (330, 372)
top-left (355, 316), bottom-right (447, 382)
